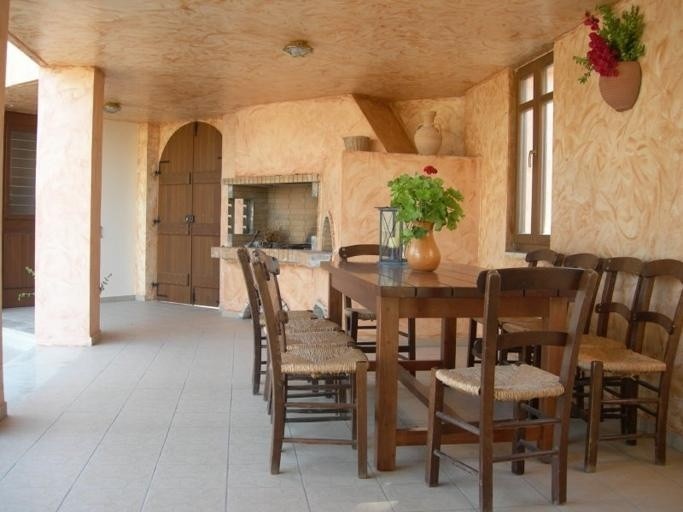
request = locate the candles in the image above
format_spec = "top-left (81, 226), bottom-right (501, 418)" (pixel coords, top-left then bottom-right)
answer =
top-left (387, 237), bottom-right (399, 249)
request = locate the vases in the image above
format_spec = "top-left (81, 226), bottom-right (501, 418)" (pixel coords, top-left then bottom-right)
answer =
top-left (404, 221), bottom-right (441, 271)
top-left (414, 109), bottom-right (443, 155)
top-left (599, 61), bottom-right (641, 110)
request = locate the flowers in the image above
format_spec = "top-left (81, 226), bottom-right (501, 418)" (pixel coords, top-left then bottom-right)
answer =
top-left (573, 3), bottom-right (645, 83)
top-left (386, 164), bottom-right (466, 243)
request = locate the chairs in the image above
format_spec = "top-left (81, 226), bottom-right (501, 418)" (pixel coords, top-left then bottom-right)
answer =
top-left (423, 267), bottom-right (598, 512)
top-left (339, 243), bottom-right (416, 380)
top-left (466, 249), bottom-right (683, 473)
top-left (237, 246), bottom-right (368, 480)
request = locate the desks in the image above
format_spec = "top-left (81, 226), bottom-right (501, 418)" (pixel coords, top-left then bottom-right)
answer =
top-left (321, 260), bottom-right (568, 472)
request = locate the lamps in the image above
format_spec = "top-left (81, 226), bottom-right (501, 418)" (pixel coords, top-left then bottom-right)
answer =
top-left (282, 40), bottom-right (314, 58)
top-left (103, 102), bottom-right (121, 114)
